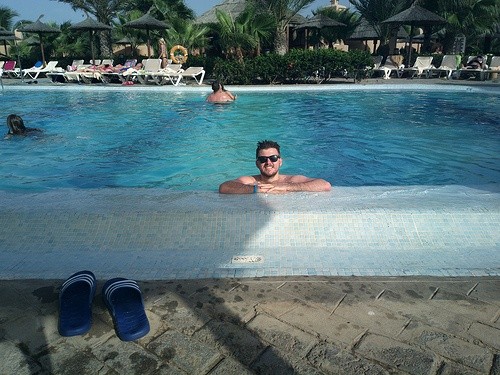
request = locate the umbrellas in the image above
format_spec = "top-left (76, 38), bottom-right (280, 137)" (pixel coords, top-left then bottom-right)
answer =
top-left (381, 4), bottom-right (448, 64)
top-left (294, 13), bottom-right (347, 47)
top-left (68, 17), bottom-right (110, 65)
top-left (17, 21), bottom-right (62, 65)
top-left (122, 13), bottom-right (170, 58)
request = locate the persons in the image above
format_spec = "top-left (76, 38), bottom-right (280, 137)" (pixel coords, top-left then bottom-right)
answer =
top-left (206, 81), bottom-right (237, 101)
top-left (82, 63), bottom-right (143, 73)
top-left (433, 47), bottom-right (442, 54)
top-left (462, 55), bottom-right (483, 69)
top-left (219, 140), bottom-right (331, 194)
top-left (7, 114), bottom-right (25, 134)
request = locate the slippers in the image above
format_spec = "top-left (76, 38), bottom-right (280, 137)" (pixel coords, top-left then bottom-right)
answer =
top-left (58, 270), bottom-right (96, 336)
top-left (102, 278), bottom-right (150, 341)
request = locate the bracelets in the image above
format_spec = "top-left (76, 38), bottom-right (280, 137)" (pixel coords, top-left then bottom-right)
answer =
top-left (254, 185), bottom-right (256, 193)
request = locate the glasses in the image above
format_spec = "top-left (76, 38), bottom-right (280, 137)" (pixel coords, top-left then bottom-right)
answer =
top-left (258, 155), bottom-right (280, 163)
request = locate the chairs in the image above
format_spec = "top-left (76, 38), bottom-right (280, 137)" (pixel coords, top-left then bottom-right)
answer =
top-left (313, 54), bottom-right (500, 81)
top-left (0, 58), bottom-right (205, 87)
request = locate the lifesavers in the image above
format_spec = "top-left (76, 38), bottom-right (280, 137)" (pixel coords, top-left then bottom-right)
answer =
top-left (169, 45), bottom-right (189, 65)
